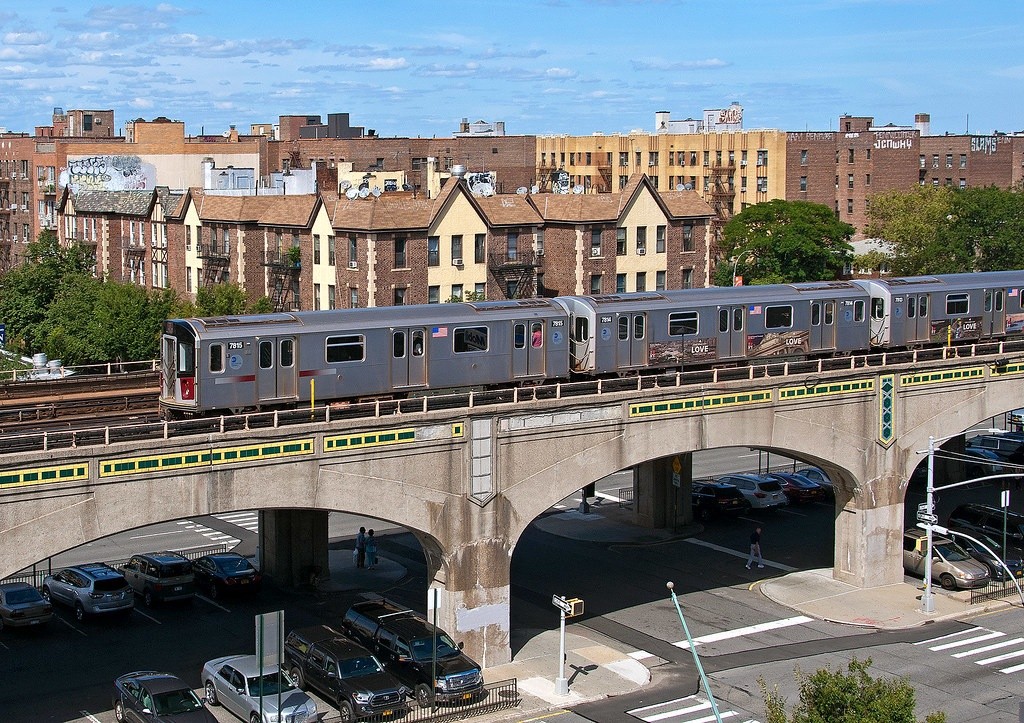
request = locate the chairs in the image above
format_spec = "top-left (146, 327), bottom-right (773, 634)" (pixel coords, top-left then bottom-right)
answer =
top-left (167, 692), bottom-right (183, 710)
top-left (143, 694), bottom-right (163, 713)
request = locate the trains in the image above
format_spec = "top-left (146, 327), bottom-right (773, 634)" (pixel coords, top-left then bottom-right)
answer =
top-left (159, 270), bottom-right (1024, 421)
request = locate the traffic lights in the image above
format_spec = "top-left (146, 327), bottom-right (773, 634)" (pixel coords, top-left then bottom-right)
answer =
top-left (571, 600), bottom-right (585, 616)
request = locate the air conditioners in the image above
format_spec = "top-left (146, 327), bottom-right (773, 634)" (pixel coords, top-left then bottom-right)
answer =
top-left (703, 161), bottom-right (709, 190)
top-left (349, 261), bottom-right (357, 268)
top-left (740, 160), bottom-right (762, 165)
top-left (185, 245), bottom-right (202, 252)
top-left (680, 160), bottom-right (686, 165)
top-left (637, 247), bottom-right (646, 255)
top-left (740, 186), bottom-right (767, 192)
top-left (622, 162), bottom-right (628, 165)
top-left (453, 258), bottom-right (464, 265)
top-left (537, 249), bottom-right (545, 256)
top-left (649, 162), bottom-right (655, 166)
top-left (592, 248), bottom-right (601, 256)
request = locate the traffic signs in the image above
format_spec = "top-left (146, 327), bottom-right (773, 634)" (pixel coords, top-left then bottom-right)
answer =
top-left (919, 502), bottom-right (935, 511)
top-left (551, 594), bottom-right (572, 614)
top-left (917, 511), bottom-right (938, 524)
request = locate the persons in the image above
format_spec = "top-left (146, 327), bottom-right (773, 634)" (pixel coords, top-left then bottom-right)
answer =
top-left (746, 527), bottom-right (765, 569)
top-left (356, 526), bottom-right (379, 570)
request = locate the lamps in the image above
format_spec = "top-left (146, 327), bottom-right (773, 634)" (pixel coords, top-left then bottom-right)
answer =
top-left (995, 357), bottom-right (1010, 367)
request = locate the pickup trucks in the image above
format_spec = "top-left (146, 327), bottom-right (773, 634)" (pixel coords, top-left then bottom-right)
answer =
top-left (342, 598), bottom-right (483, 709)
top-left (283, 624), bottom-right (407, 723)
top-left (950, 502), bottom-right (1024, 546)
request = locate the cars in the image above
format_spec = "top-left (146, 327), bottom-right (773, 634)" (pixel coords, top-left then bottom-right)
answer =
top-left (112, 670), bottom-right (220, 723)
top-left (200, 654), bottom-right (318, 723)
top-left (0, 582), bottom-right (53, 629)
top-left (946, 527), bottom-right (1024, 582)
top-left (764, 466), bottom-right (835, 507)
top-left (191, 552), bottom-right (260, 600)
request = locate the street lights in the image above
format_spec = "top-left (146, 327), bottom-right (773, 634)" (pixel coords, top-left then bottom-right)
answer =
top-left (920, 428), bottom-right (999, 611)
top-left (732, 250), bottom-right (753, 286)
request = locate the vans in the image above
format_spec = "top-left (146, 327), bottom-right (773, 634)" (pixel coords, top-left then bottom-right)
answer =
top-left (903, 529), bottom-right (991, 590)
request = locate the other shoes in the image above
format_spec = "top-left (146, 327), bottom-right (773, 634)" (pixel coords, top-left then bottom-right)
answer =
top-left (746, 565), bottom-right (750, 569)
top-left (758, 565), bottom-right (764, 568)
top-left (368, 567), bottom-right (371, 570)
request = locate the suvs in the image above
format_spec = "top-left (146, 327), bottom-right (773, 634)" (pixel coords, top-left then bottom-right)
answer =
top-left (42, 562), bottom-right (136, 620)
top-left (691, 472), bottom-right (787, 523)
top-left (119, 550), bottom-right (198, 608)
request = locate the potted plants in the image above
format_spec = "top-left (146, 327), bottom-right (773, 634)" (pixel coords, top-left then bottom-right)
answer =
top-left (284, 243), bottom-right (302, 267)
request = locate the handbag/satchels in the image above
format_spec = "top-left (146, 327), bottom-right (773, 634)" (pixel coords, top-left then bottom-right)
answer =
top-left (375, 557), bottom-right (378, 564)
top-left (353, 551), bottom-right (357, 563)
